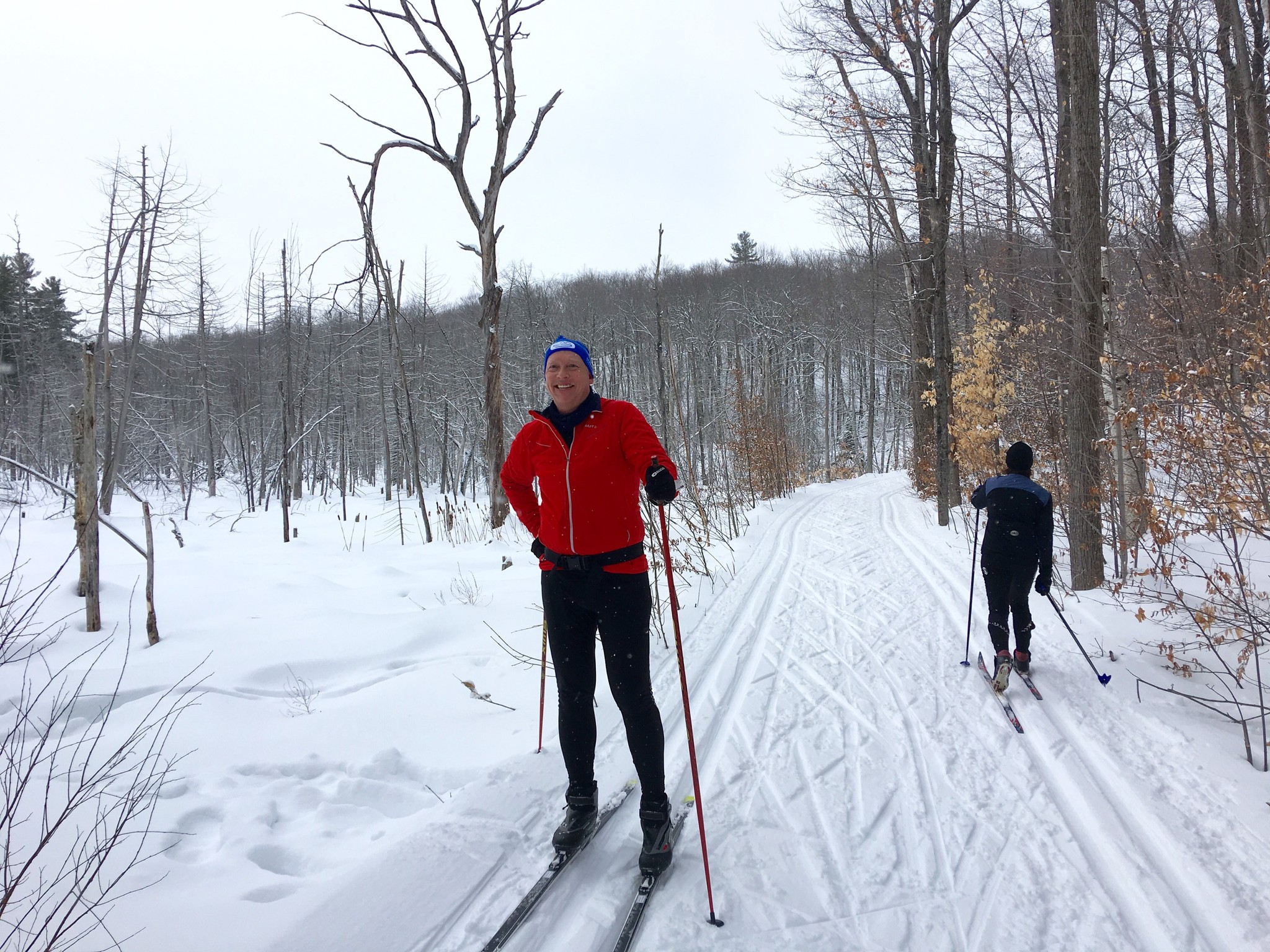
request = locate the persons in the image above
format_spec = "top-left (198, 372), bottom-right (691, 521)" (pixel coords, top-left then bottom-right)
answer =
top-left (499, 335), bottom-right (685, 877)
top-left (971, 441), bottom-right (1054, 691)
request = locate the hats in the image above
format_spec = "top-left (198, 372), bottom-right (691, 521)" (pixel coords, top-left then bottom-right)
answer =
top-left (543, 335), bottom-right (592, 377)
top-left (1005, 441), bottom-right (1034, 469)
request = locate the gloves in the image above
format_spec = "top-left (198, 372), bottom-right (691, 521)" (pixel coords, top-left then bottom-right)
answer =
top-left (530, 536), bottom-right (546, 558)
top-left (644, 464), bottom-right (677, 507)
top-left (1033, 573), bottom-right (1053, 596)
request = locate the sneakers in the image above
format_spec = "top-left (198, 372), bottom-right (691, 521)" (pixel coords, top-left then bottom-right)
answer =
top-left (1013, 647), bottom-right (1031, 673)
top-left (551, 781), bottom-right (599, 850)
top-left (638, 793), bottom-right (674, 876)
top-left (991, 654), bottom-right (1014, 693)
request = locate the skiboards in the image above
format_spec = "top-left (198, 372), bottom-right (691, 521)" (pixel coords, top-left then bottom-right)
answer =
top-left (977, 650), bottom-right (1044, 734)
top-left (478, 778), bottom-right (701, 952)
